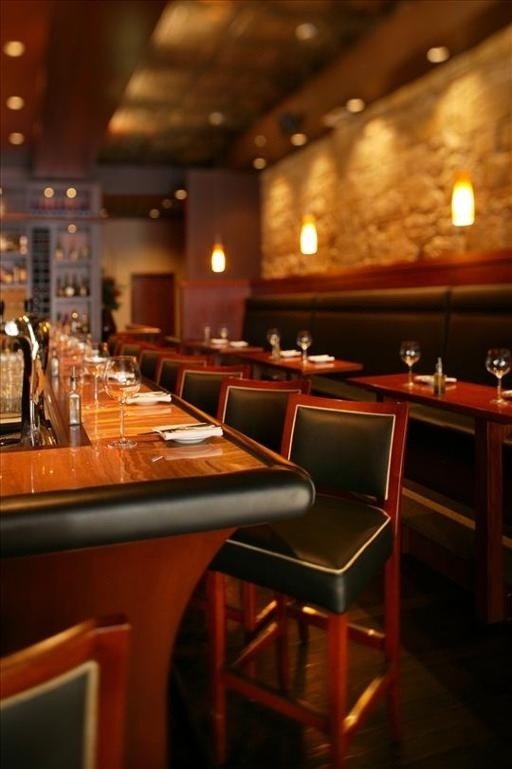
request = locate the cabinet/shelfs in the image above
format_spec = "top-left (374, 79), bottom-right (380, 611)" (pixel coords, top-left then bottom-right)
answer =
top-left (1, 177), bottom-right (107, 342)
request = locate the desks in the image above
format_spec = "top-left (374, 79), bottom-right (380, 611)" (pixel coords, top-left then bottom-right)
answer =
top-left (346, 366), bottom-right (511, 624)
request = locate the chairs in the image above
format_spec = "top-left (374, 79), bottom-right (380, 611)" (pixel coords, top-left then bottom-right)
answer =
top-left (110, 343), bottom-right (250, 420)
top-left (1, 612), bottom-right (132, 769)
top-left (191, 377), bottom-right (313, 697)
top-left (203, 393), bottom-right (410, 768)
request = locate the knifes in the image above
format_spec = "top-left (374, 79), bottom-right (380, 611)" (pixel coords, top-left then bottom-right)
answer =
top-left (137, 423), bottom-right (213, 436)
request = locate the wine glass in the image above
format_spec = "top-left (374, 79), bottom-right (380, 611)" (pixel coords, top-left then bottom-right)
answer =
top-left (486, 350), bottom-right (511, 408)
top-left (400, 341), bottom-right (420, 389)
top-left (203, 327), bottom-right (336, 364)
top-left (42, 337), bottom-right (111, 409)
top-left (104, 356), bottom-right (143, 449)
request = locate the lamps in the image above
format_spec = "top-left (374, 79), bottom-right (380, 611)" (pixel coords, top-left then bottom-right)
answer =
top-left (451, 176), bottom-right (474, 226)
top-left (211, 230), bottom-right (225, 276)
top-left (300, 210), bottom-right (318, 254)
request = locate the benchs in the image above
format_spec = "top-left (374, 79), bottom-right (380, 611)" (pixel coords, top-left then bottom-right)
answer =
top-left (242, 286), bottom-right (511, 495)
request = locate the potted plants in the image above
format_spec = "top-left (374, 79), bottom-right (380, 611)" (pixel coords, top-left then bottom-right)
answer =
top-left (101, 270), bottom-right (123, 343)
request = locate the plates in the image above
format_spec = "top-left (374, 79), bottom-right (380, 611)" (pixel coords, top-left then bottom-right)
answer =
top-left (174, 438), bottom-right (206, 445)
top-left (416, 374), bottom-right (456, 385)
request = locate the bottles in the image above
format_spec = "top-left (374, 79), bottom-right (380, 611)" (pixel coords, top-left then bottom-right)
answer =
top-left (433, 357), bottom-right (450, 395)
top-left (2, 227), bottom-right (91, 336)
top-left (63, 376), bottom-right (81, 427)
